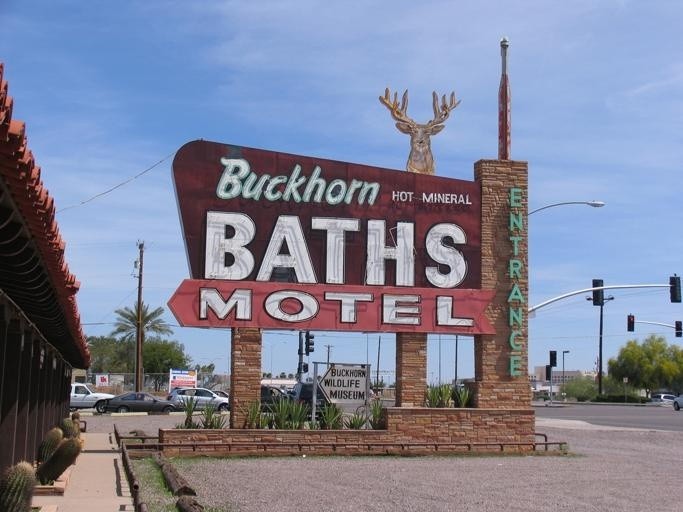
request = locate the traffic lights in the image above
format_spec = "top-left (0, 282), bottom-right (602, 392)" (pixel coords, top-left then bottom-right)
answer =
top-left (669, 276), bottom-right (681, 302)
top-left (676, 321), bottom-right (682, 337)
top-left (303, 364), bottom-right (308, 373)
top-left (627, 315), bottom-right (634, 331)
top-left (306, 331), bottom-right (314, 356)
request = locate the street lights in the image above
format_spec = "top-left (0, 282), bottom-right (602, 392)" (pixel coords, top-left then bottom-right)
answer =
top-left (586, 295), bottom-right (615, 398)
top-left (528, 200), bottom-right (605, 215)
top-left (562, 350), bottom-right (569, 398)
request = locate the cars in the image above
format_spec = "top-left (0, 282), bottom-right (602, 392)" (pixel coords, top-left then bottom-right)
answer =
top-left (672, 392), bottom-right (683, 411)
top-left (69, 380), bottom-right (330, 419)
top-left (652, 394), bottom-right (677, 404)
